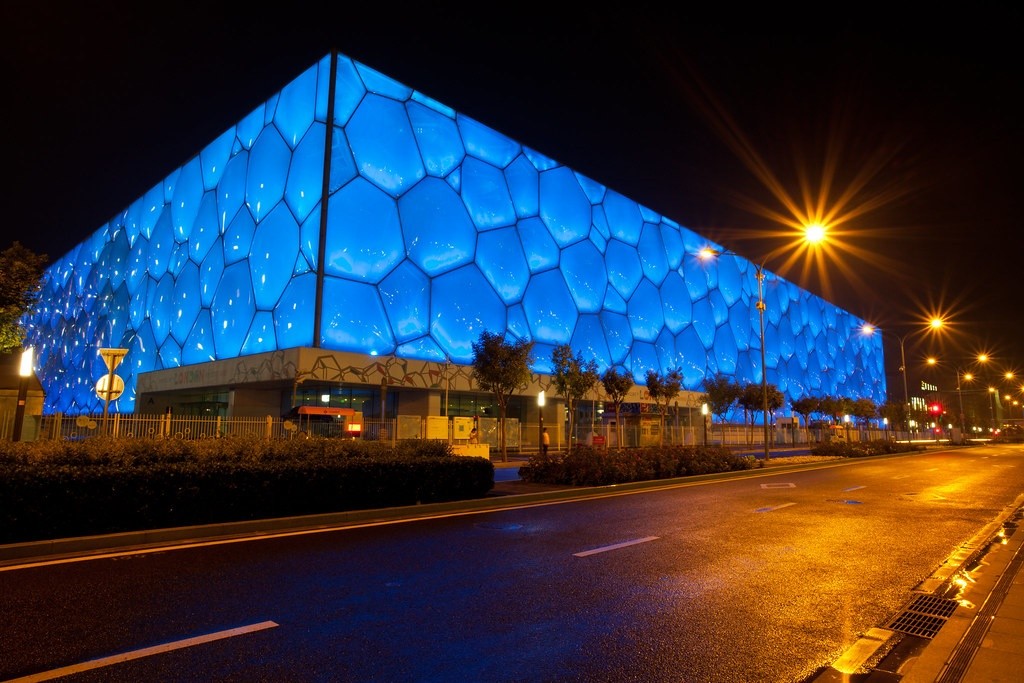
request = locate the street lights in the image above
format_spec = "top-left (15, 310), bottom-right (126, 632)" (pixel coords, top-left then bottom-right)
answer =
top-left (12, 346), bottom-right (35, 442)
top-left (924, 351), bottom-right (1024, 444)
top-left (698, 222), bottom-right (827, 461)
top-left (537, 390), bottom-right (546, 454)
top-left (911, 419), bottom-right (915, 441)
top-left (862, 315), bottom-right (945, 444)
top-left (844, 415), bottom-right (850, 444)
top-left (884, 417), bottom-right (888, 442)
top-left (701, 403), bottom-right (709, 447)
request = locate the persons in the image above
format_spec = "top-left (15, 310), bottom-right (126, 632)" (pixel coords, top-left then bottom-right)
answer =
top-left (542, 428), bottom-right (550, 454)
top-left (469, 428), bottom-right (479, 444)
